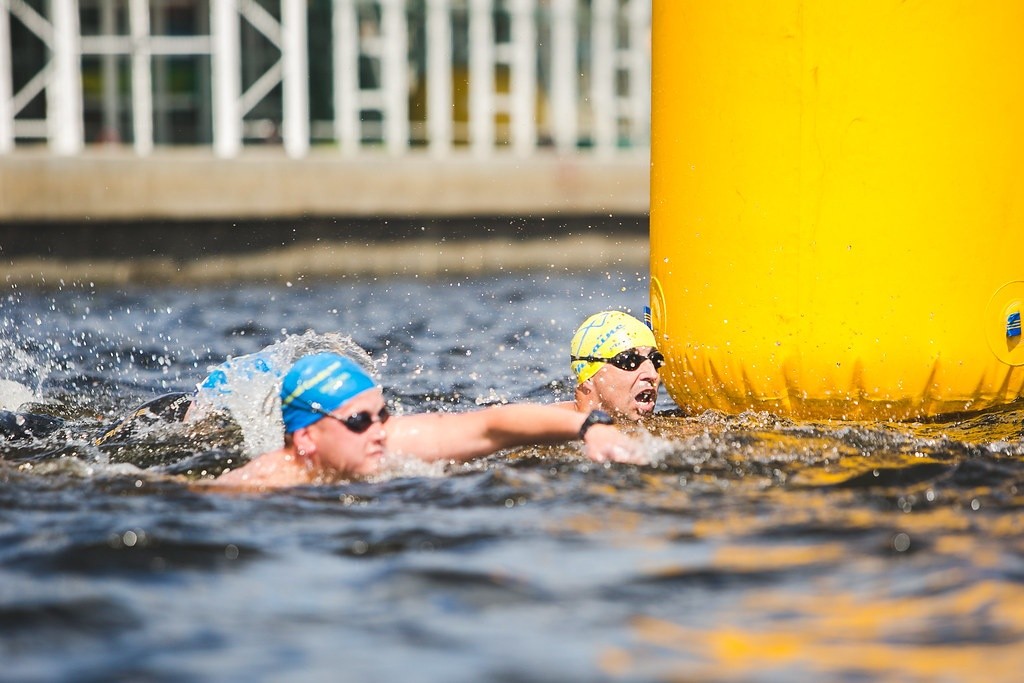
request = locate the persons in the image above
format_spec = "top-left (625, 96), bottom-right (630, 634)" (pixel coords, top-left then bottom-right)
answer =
top-left (543, 310), bottom-right (664, 428)
top-left (210, 348), bottom-right (660, 490)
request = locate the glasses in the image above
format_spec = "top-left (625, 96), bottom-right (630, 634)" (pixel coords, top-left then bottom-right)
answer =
top-left (571, 350), bottom-right (665, 371)
top-left (282, 389), bottom-right (389, 433)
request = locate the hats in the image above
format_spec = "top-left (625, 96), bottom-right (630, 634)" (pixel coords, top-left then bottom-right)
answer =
top-left (571, 310), bottom-right (658, 385)
top-left (281, 352), bottom-right (377, 433)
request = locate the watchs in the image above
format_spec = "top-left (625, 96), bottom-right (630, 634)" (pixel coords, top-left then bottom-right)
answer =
top-left (577, 407), bottom-right (615, 442)
